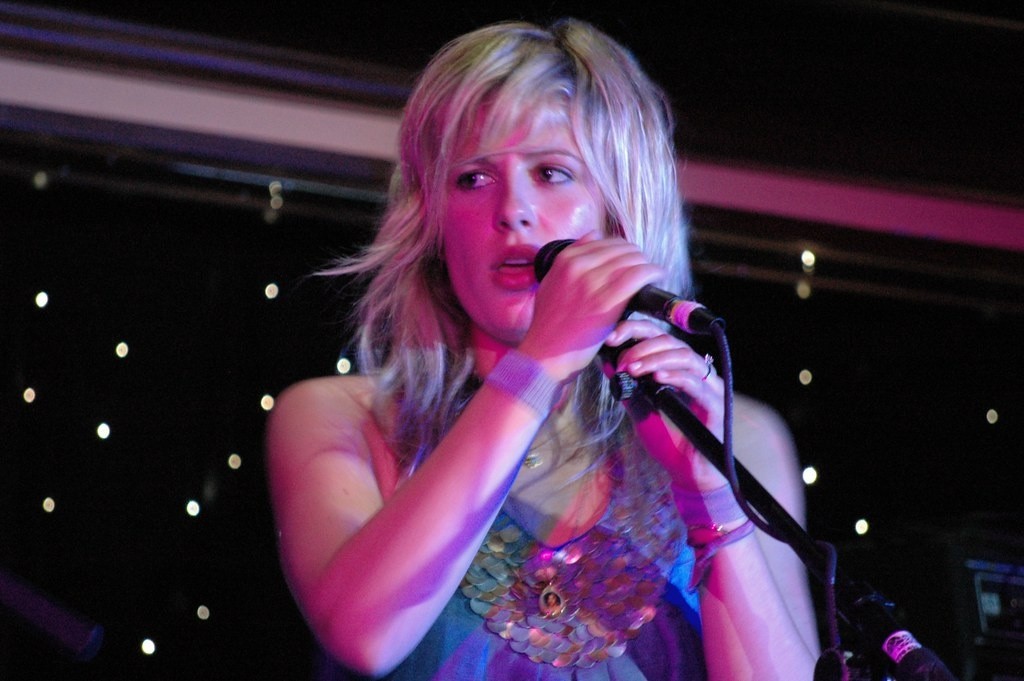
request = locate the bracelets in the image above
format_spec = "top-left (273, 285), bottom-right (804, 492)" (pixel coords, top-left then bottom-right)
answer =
top-left (687, 520), bottom-right (756, 591)
top-left (670, 482), bottom-right (745, 530)
top-left (485, 349), bottom-right (564, 421)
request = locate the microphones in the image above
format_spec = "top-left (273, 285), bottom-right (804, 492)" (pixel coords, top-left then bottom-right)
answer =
top-left (534, 239), bottom-right (726, 335)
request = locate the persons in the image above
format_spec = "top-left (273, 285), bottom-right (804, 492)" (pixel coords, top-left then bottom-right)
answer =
top-left (269, 21), bottom-right (820, 681)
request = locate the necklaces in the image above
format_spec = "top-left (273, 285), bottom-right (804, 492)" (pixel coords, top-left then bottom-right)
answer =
top-left (523, 417), bottom-right (579, 469)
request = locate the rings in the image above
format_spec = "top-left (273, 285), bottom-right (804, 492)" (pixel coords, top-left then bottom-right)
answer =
top-left (702, 354), bottom-right (714, 381)
